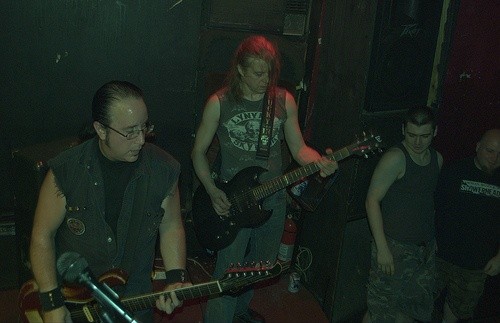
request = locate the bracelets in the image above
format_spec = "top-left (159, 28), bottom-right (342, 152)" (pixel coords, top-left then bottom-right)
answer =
top-left (38, 286), bottom-right (68, 313)
top-left (165, 268), bottom-right (187, 287)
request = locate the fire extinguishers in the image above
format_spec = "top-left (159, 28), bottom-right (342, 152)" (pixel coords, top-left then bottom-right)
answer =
top-left (277, 214), bottom-right (297, 262)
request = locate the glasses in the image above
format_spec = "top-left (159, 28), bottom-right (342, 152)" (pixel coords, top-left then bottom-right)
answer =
top-left (98, 120), bottom-right (155, 139)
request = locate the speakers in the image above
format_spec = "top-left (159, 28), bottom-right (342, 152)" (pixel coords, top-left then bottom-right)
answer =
top-left (195, 0), bottom-right (460, 323)
top-left (0, 233), bottom-right (21, 289)
top-left (9, 124), bottom-right (95, 222)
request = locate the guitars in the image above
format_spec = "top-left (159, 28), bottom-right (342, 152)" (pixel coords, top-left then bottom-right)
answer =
top-left (189, 129), bottom-right (385, 250)
top-left (19, 259), bottom-right (284, 323)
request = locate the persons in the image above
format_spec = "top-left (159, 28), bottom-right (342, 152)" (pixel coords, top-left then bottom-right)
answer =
top-left (363, 107), bottom-right (443, 323)
top-left (30, 80), bottom-right (187, 322)
top-left (191, 35), bottom-right (340, 322)
top-left (433, 129), bottom-right (500, 323)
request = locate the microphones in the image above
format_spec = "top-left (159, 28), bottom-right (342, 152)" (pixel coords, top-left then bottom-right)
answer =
top-left (56, 252), bottom-right (141, 322)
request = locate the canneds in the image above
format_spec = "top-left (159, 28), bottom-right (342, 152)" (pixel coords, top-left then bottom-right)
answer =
top-left (288, 272), bottom-right (300, 293)
